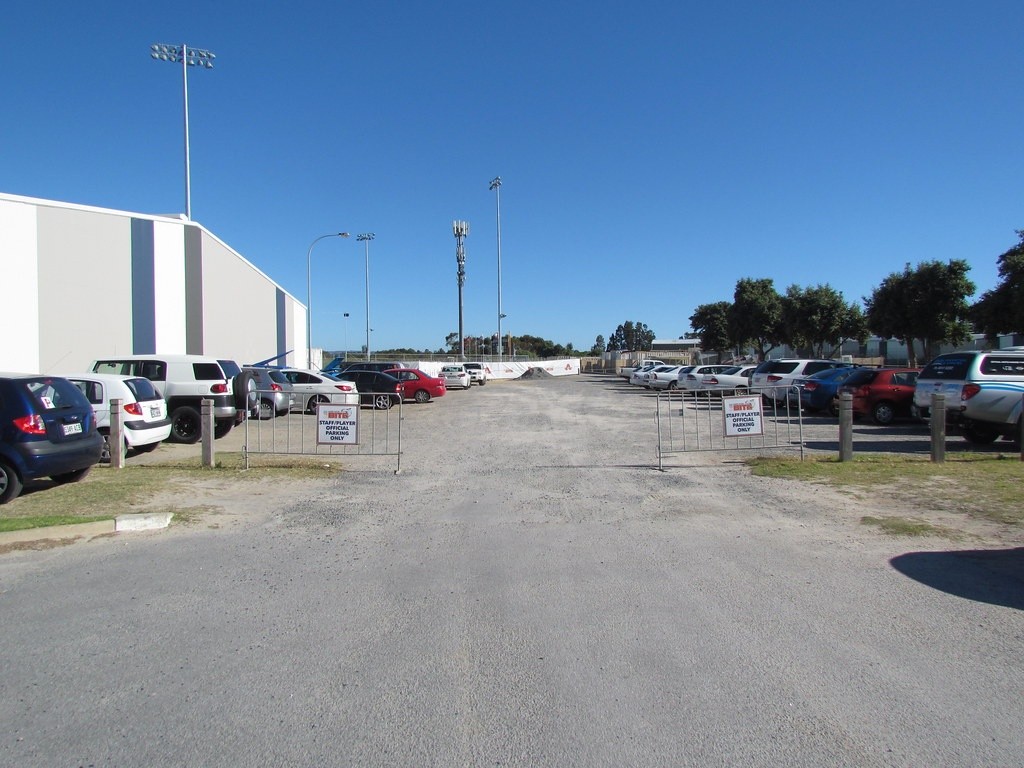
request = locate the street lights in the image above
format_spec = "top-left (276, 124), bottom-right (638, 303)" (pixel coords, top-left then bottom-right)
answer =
top-left (307, 232), bottom-right (350, 369)
top-left (356, 232), bottom-right (375, 359)
top-left (489, 175), bottom-right (503, 359)
top-left (150, 43), bottom-right (217, 221)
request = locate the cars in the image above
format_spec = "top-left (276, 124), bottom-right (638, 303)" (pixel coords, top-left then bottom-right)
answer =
top-left (239, 366), bottom-right (297, 420)
top-left (798, 366), bottom-right (864, 417)
top-left (440, 364), bottom-right (472, 390)
top-left (382, 368), bottom-right (446, 404)
top-left (278, 369), bottom-right (360, 416)
top-left (834, 367), bottom-right (924, 427)
top-left (620, 359), bottom-right (760, 401)
top-left (320, 361), bottom-right (409, 410)
top-left (0, 371), bottom-right (104, 504)
top-left (32, 372), bottom-right (172, 464)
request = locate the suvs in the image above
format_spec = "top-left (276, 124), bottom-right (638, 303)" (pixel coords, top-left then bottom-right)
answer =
top-left (750, 358), bottom-right (845, 410)
top-left (79, 354), bottom-right (260, 444)
top-left (913, 346), bottom-right (1024, 454)
top-left (460, 362), bottom-right (488, 385)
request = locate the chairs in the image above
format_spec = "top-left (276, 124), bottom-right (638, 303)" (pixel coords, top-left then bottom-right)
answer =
top-left (150, 366), bottom-right (165, 381)
